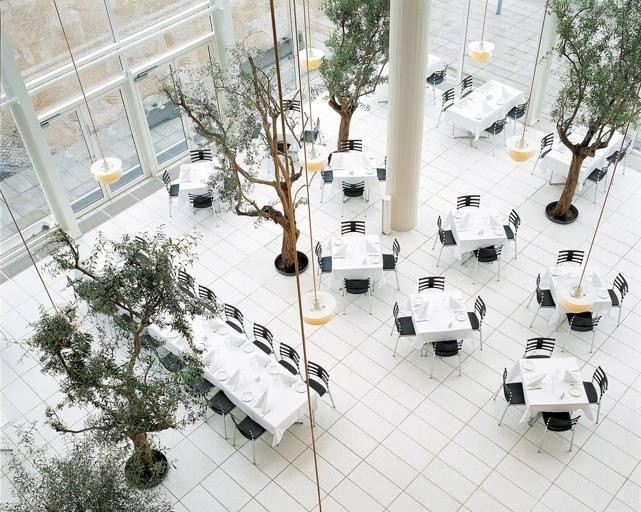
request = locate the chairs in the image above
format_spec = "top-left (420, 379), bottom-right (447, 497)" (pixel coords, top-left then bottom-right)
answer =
top-left (314, 220), bottom-right (400, 316)
top-left (319, 138), bottom-right (387, 219)
top-left (530, 117), bottom-right (634, 205)
top-left (245, 97), bottom-right (321, 167)
top-left (425, 52), bottom-right (531, 150)
top-left (70, 234), bottom-right (336, 456)
top-left (431, 194), bottom-right (521, 282)
top-left (526, 249), bottom-right (629, 353)
top-left (162, 148), bottom-right (236, 229)
top-left (390, 276), bottom-right (487, 380)
top-left (493, 335), bottom-right (610, 453)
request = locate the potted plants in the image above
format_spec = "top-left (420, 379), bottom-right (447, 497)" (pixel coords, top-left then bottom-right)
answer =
top-left (151, 30), bottom-right (327, 301)
top-left (7, 222), bottom-right (219, 498)
top-left (541, 0), bottom-right (641, 234)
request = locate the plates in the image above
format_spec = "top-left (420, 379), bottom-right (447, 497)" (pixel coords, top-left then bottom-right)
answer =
top-left (369, 256), bottom-right (381, 264)
top-left (334, 238), bottom-right (343, 246)
top-left (551, 267), bottom-right (561, 276)
top-left (217, 327), bottom-right (229, 334)
top-left (568, 387), bottom-right (582, 397)
top-left (242, 343), bottom-right (308, 393)
top-left (596, 288), bottom-right (609, 299)
top-left (414, 296), bottom-right (424, 305)
top-left (495, 229), bottom-right (505, 235)
top-left (217, 370), bottom-right (254, 401)
top-left (524, 360), bottom-right (535, 370)
top-left (455, 312), bottom-right (466, 321)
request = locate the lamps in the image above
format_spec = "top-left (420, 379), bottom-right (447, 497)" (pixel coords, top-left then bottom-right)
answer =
top-left (555, 83), bottom-right (641, 315)
top-left (507, 0), bottom-right (549, 163)
top-left (53, 4), bottom-right (124, 185)
top-left (469, 0), bottom-right (497, 63)
top-left (286, 1), bottom-right (338, 327)
